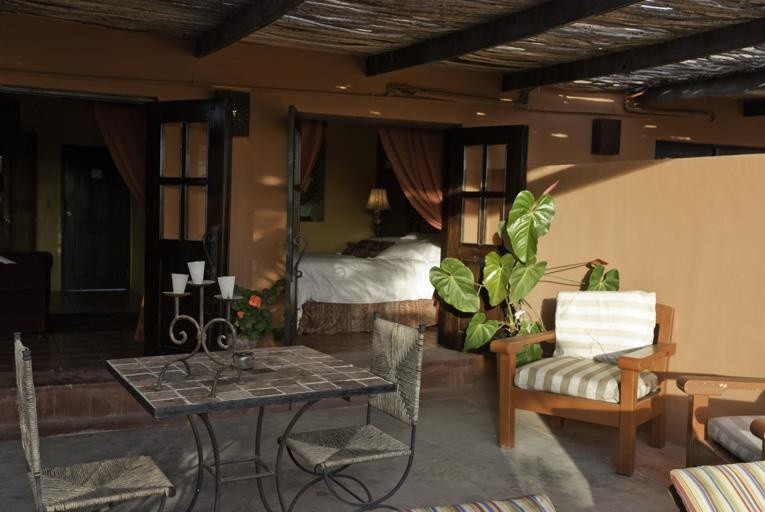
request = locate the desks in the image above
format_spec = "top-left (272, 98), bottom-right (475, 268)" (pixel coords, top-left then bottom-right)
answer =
top-left (105, 343), bottom-right (395, 511)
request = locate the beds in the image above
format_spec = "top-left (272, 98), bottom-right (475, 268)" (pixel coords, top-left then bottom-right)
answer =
top-left (292, 229), bottom-right (443, 335)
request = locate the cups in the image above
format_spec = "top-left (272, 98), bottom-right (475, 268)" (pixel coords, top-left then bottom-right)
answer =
top-left (186, 261), bottom-right (206, 284)
top-left (232, 352), bottom-right (255, 369)
top-left (171, 274), bottom-right (189, 294)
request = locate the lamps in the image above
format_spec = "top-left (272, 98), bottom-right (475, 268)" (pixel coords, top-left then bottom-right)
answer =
top-left (361, 187), bottom-right (392, 237)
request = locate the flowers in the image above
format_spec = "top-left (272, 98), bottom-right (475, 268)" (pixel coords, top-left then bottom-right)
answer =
top-left (228, 279), bottom-right (284, 342)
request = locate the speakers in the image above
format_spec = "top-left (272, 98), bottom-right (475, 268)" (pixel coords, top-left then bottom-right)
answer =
top-left (215, 89), bottom-right (250, 137)
top-left (591, 119), bottom-right (621, 155)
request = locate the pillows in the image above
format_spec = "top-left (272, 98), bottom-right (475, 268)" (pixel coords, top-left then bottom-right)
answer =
top-left (340, 235), bottom-right (442, 264)
top-left (402, 491), bottom-right (555, 510)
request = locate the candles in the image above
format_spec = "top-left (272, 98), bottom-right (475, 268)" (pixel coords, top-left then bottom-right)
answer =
top-left (170, 272), bottom-right (190, 292)
top-left (186, 261), bottom-right (205, 287)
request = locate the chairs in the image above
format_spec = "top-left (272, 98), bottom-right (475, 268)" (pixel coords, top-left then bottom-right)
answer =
top-left (10, 330), bottom-right (176, 510)
top-left (666, 458), bottom-right (765, 512)
top-left (488, 288), bottom-right (678, 478)
top-left (275, 306), bottom-right (428, 511)
top-left (0, 250), bottom-right (54, 338)
top-left (676, 374), bottom-right (765, 469)
